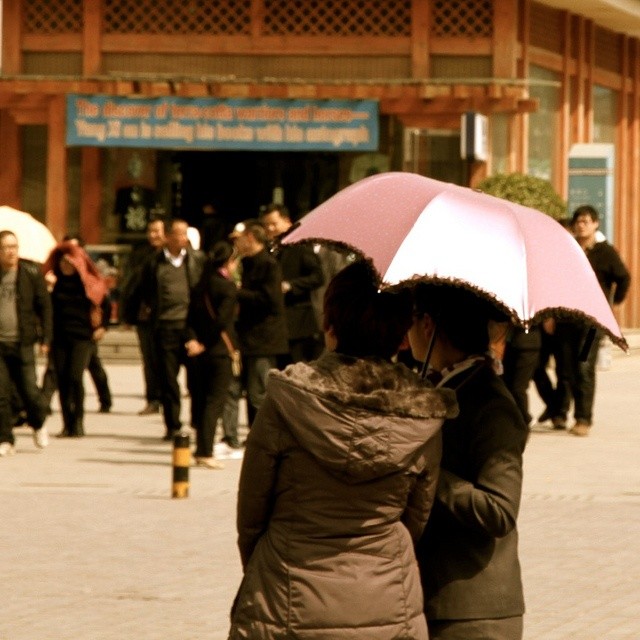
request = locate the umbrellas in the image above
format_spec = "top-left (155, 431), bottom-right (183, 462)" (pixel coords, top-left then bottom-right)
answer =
top-left (281, 170), bottom-right (629, 348)
top-left (0, 207), bottom-right (60, 266)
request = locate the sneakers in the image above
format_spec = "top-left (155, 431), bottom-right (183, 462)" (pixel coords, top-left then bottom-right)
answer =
top-left (58, 426), bottom-right (72, 436)
top-left (33, 427), bottom-right (48, 447)
top-left (0, 442), bottom-right (16, 456)
top-left (540, 407), bottom-right (551, 421)
top-left (571, 423), bottom-right (589, 436)
top-left (99, 400), bottom-right (112, 413)
top-left (196, 457), bottom-right (224, 470)
top-left (73, 426), bottom-right (83, 436)
top-left (163, 420), bottom-right (181, 441)
top-left (138, 401), bottom-right (159, 415)
top-left (553, 416), bottom-right (564, 431)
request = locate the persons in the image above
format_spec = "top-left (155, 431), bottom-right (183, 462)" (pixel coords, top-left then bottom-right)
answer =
top-left (405, 283), bottom-right (525, 639)
top-left (39, 245), bottom-right (111, 436)
top-left (259, 203), bottom-right (325, 370)
top-left (0, 231), bottom-right (51, 455)
top-left (220, 221), bottom-right (257, 447)
top-left (131, 218), bottom-right (207, 442)
top-left (554, 206), bottom-right (630, 436)
top-left (120, 217), bottom-right (166, 416)
top-left (184, 243), bottom-right (241, 468)
top-left (234, 224), bottom-right (288, 447)
top-left (229, 261), bottom-right (459, 639)
top-left (41, 233), bottom-right (113, 412)
top-left (502, 317), bottom-right (541, 426)
top-left (532, 217), bottom-right (575, 423)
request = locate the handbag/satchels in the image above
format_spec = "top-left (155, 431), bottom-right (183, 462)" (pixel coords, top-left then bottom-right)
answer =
top-left (230, 349), bottom-right (242, 377)
